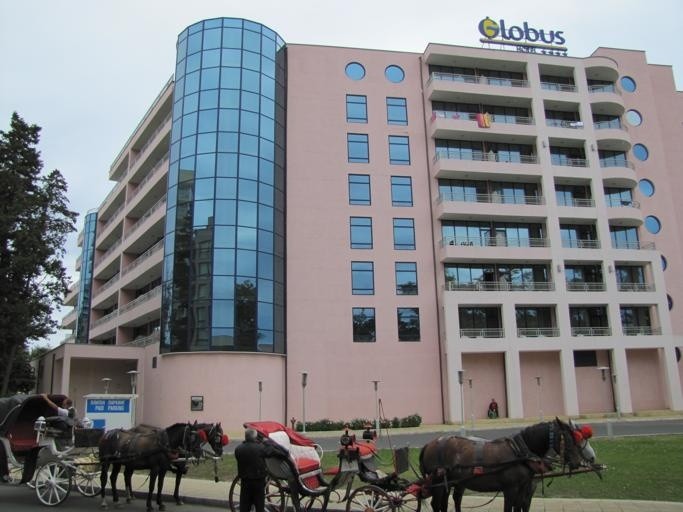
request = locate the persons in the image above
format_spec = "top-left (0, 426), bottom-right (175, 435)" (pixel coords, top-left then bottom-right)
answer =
top-left (488, 398), bottom-right (498, 417)
top-left (234, 429), bottom-right (280, 512)
top-left (41, 393), bottom-right (77, 417)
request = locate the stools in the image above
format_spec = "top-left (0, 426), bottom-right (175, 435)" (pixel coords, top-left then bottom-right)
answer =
top-left (339, 440), bottom-right (392, 483)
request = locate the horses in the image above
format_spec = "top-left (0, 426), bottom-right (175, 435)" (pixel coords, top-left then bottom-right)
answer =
top-left (418, 415), bottom-right (582, 512)
top-left (123, 422), bottom-right (224, 506)
top-left (452, 417), bottom-right (597, 512)
top-left (98, 419), bottom-right (204, 512)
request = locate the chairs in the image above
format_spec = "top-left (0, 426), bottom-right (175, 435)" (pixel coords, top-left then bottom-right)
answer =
top-left (242, 421), bottom-right (329, 496)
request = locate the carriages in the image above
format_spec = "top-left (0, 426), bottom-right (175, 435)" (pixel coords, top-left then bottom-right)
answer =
top-left (226, 416), bottom-right (608, 512)
top-left (0, 390), bottom-right (229, 512)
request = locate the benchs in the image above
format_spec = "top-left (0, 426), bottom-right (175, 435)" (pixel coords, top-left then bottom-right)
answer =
top-left (0, 394), bottom-right (67, 453)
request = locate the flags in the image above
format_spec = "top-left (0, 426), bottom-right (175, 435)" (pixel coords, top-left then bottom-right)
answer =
top-left (477, 112), bottom-right (491, 128)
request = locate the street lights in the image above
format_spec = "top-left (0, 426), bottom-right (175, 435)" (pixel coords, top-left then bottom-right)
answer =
top-left (100, 377), bottom-right (112, 394)
top-left (127, 370), bottom-right (140, 394)
